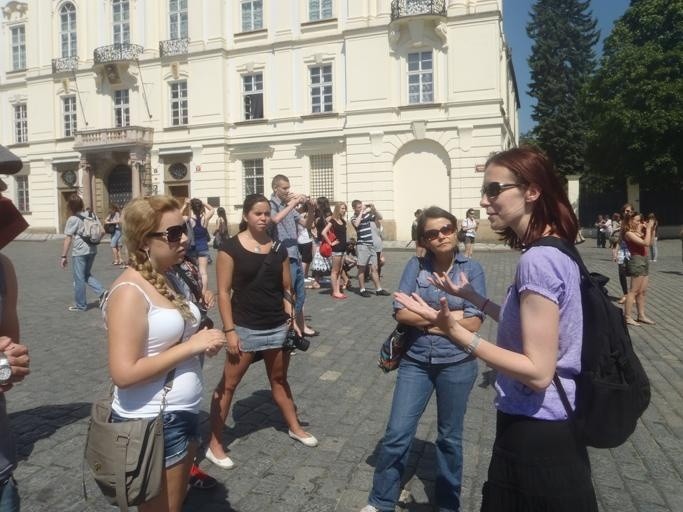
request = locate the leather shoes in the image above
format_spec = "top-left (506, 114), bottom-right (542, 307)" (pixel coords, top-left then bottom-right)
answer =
top-left (289, 428), bottom-right (319, 446)
top-left (201, 445), bottom-right (234, 470)
top-left (332, 278), bottom-right (390, 299)
top-left (302, 330), bottom-right (319, 338)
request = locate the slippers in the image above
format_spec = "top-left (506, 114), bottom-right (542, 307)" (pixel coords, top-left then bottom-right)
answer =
top-left (626, 318), bottom-right (640, 326)
top-left (638, 315), bottom-right (656, 325)
top-left (619, 294), bottom-right (626, 304)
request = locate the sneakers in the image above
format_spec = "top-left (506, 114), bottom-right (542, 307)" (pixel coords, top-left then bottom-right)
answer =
top-left (304, 276), bottom-right (331, 289)
top-left (186, 465), bottom-right (218, 489)
top-left (359, 505), bottom-right (379, 512)
top-left (98, 291), bottom-right (109, 309)
top-left (69, 304), bottom-right (86, 311)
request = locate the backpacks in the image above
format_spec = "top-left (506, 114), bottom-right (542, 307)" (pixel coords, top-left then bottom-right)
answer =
top-left (522, 237), bottom-right (652, 450)
top-left (74, 210), bottom-right (102, 245)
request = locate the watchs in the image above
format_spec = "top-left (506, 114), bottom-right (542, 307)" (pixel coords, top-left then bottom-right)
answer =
top-left (0, 351), bottom-right (12, 386)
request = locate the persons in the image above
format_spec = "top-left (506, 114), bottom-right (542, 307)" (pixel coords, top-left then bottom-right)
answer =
top-left (360, 206), bottom-right (486, 512)
top-left (0, 144), bottom-right (32, 511)
top-left (62, 174), bottom-right (392, 511)
top-left (412, 208), bottom-right (480, 258)
top-left (393, 142), bottom-right (598, 511)
top-left (575, 203), bottom-right (658, 327)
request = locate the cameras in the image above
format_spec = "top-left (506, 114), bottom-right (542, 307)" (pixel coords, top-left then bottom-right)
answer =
top-left (283, 329), bottom-right (310, 355)
top-left (646, 218), bottom-right (658, 228)
top-left (192, 301), bottom-right (213, 333)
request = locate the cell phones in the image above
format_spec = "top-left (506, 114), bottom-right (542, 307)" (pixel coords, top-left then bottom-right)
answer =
top-left (364, 203), bottom-right (372, 209)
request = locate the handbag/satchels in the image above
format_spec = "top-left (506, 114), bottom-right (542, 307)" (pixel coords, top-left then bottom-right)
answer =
top-left (218, 222), bottom-right (228, 236)
top-left (83, 396), bottom-right (165, 504)
top-left (457, 229), bottom-right (466, 241)
top-left (103, 223), bottom-right (115, 234)
top-left (622, 258), bottom-right (630, 277)
top-left (378, 326), bottom-right (409, 373)
top-left (319, 234), bottom-right (335, 259)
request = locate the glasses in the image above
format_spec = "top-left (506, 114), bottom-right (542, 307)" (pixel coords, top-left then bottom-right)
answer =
top-left (422, 223), bottom-right (456, 240)
top-left (146, 222), bottom-right (188, 243)
top-left (481, 182), bottom-right (527, 200)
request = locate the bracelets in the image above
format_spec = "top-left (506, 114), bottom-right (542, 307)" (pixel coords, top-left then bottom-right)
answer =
top-left (481, 298), bottom-right (489, 311)
top-left (463, 332), bottom-right (481, 354)
